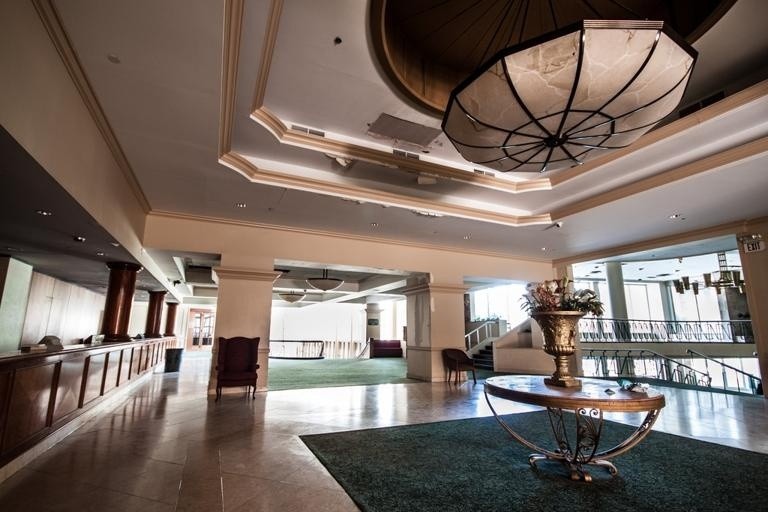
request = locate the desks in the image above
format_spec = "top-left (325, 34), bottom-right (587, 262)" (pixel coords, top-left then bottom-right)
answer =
top-left (484, 375), bottom-right (665, 484)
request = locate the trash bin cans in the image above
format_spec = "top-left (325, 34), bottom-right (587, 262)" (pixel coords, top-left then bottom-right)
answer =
top-left (165, 348), bottom-right (184, 372)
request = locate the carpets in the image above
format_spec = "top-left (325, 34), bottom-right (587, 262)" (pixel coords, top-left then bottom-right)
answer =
top-left (298, 409), bottom-right (768, 512)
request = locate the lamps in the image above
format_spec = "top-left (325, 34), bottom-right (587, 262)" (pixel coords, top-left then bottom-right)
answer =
top-left (672, 251), bottom-right (745, 296)
top-left (306, 269), bottom-right (344, 292)
top-left (441, 1), bottom-right (700, 174)
top-left (279, 290), bottom-right (306, 303)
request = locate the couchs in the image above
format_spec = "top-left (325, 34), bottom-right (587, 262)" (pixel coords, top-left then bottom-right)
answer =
top-left (370, 338), bottom-right (402, 358)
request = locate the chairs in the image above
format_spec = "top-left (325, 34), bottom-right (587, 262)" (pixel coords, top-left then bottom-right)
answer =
top-left (216, 336), bottom-right (260, 403)
top-left (443, 349), bottom-right (477, 387)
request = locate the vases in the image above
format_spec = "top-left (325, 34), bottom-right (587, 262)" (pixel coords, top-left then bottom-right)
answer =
top-left (530, 310), bottom-right (587, 387)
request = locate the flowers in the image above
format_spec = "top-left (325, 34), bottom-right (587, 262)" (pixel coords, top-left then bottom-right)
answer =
top-left (520, 275), bottom-right (606, 317)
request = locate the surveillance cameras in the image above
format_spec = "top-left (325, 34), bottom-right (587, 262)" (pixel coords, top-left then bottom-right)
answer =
top-left (557, 222), bottom-right (562, 228)
